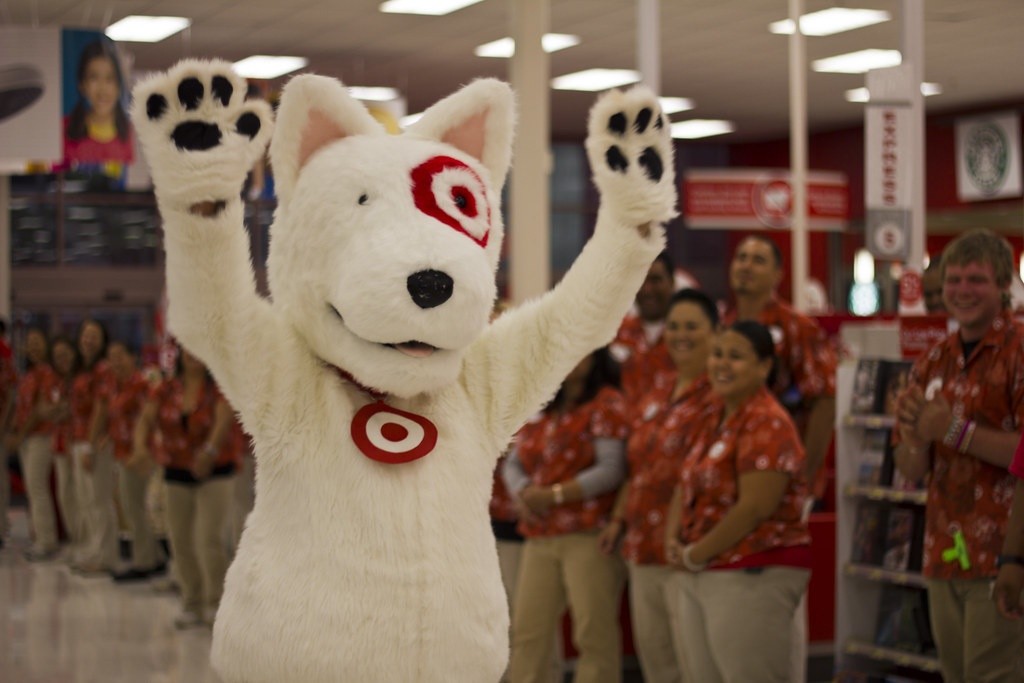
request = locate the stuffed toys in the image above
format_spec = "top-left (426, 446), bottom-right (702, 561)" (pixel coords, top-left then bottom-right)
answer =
top-left (133, 58), bottom-right (679, 683)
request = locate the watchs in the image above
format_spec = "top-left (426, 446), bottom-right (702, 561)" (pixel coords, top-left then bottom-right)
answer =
top-left (995, 553), bottom-right (1024, 569)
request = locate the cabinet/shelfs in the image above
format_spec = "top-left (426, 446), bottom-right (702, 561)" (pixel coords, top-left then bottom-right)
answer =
top-left (837, 323), bottom-right (979, 672)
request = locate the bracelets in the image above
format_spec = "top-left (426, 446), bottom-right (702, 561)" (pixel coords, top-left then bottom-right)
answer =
top-left (553, 484), bottom-right (563, 503)
top-left (955, 420), bottom-right (970, 449)
top-left (202, 442), bottom-right (218, 455)
top-left (943, 417), bottom-right (977, 455)
top-left (683, 545), bottom-right (705, 572)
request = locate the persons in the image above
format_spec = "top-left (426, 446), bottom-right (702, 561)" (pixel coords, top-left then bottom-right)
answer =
top-left (720, 236), bottom-right (836, 484)
top-left (890, 225), bottom-right (1024, 683)
top-left (0, 314), bottom-right (260, 630)
top-left (489, 255), bottom-right (812, 683)
top-left (64, 43), bottom-right (137, 181)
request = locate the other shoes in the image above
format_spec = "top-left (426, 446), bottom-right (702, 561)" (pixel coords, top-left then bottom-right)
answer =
top-left (24, 542), bottom-right (59, 563)
top-left (155, 580), bottom-right (178, 593)
top-left (174, 610), bottom-right (199, 627)
